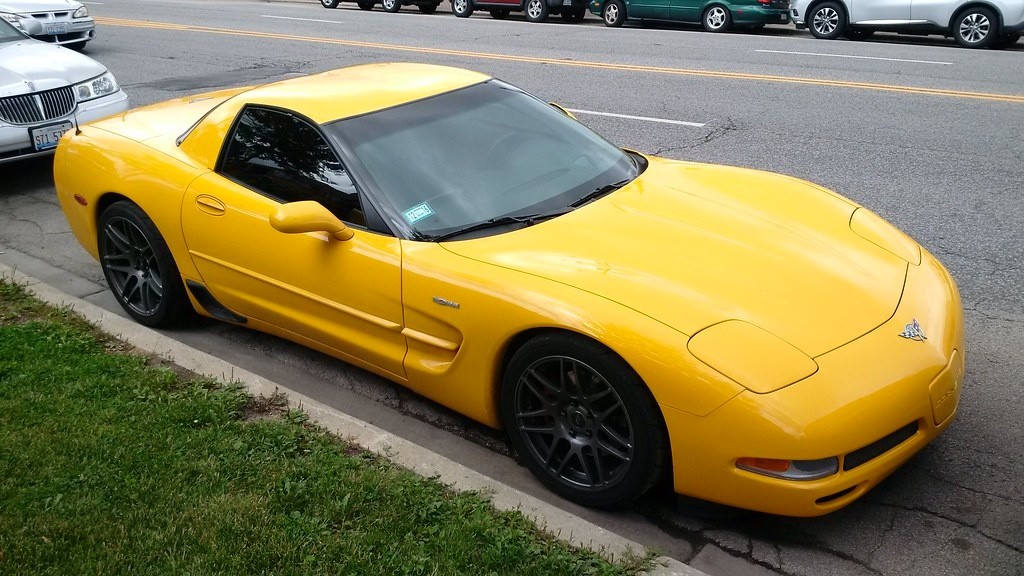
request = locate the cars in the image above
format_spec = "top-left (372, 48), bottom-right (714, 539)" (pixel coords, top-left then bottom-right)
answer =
top-left (0, 0), bottom-right (129, 168)
top-left (790, 0), bottom-right (1024, 49)
top-left (319, 0), bottom-right (444, 12)
top-left (449, 0), bottom-right (588, 23)
top-left (589, 0), bottom-right (791, 33)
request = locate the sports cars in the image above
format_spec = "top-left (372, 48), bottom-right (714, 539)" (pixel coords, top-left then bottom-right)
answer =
top-left (52, 62), bottom-right (966, 516)
